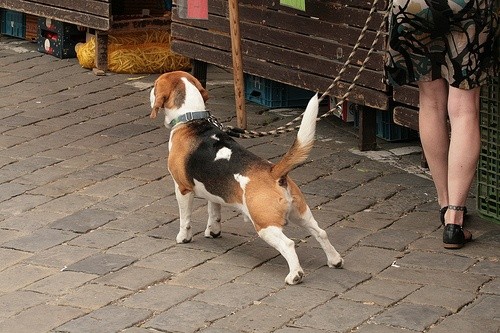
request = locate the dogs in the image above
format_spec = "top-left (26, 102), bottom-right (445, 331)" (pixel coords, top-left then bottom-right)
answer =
top-left (149, 71), bottom-right (344, 285)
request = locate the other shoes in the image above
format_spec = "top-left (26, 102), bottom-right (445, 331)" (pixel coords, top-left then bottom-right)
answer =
top-left (439, 206), bottom-right (449, 225)
top-left (443, 224), bottom-right (473, 250)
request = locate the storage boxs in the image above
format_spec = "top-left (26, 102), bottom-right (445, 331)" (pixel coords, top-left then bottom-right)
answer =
top-left (25, 14), bottom-right (38, 42)
top-left (5, 11), bottom-right (26, 38)
top-left (328, 96), bottom-right (355, 122)
top-left (36, 17), bottom-right (87, 59)
top-left (243, 73), bottom-right (328, 108)
top-left (0, 9), bottom-right (6, 34)
top-left (476, 61), bottom-right (500, 224)
top-left (354, 105), bottom-right (418, 142)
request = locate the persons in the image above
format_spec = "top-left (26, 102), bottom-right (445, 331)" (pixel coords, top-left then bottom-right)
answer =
top-left (383, 0), bottom-right (500, 248)
